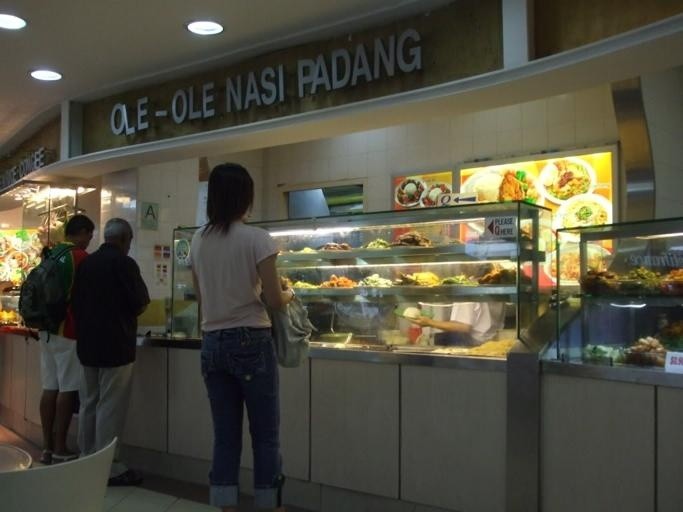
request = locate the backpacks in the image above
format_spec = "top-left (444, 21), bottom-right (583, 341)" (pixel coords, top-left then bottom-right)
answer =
top-left (16, 242), bottom-right (81, 333)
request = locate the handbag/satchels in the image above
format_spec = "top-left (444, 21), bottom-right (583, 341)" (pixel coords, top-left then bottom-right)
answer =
top-left (259, 273), bottom-right (316, 369)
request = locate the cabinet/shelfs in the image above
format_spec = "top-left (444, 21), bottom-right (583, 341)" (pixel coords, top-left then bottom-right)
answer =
top-left (553, 216), bottom-right (683, 374)
top-left (169, 200), bottom-right (545, 360)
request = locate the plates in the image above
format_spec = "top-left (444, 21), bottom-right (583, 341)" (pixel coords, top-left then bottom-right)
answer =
top-left (419, 182), bottom-right (451, 208)
top-left (457, 157), bottom-right (612, 286)
top-left (288, 243), bottom-right (440, 261)
top-left (394, 176), bottom-right (426, 207)
top-left (393, 308), bottom-right (436, 321)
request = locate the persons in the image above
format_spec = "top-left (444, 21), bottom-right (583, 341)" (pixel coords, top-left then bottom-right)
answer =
top-left (408, 302), bottom-right (505, 348)
top-left (19, 215), bottom-right (95, 464)
top-left (190, 162), bottom-right (296, 511)
top-left (71, 218), bottom-right (150, 485)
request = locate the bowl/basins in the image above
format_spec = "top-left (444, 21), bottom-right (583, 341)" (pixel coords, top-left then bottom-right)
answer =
top-left (620, 345), bottom-right (665, 369)
top-left (320, 333), bottom-right (352, 344)
top-left (578, 278), bottom-right (683, 297)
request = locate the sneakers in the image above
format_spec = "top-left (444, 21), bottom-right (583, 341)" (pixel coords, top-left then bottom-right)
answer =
top-left (39, 447), bottom-right (144, 487)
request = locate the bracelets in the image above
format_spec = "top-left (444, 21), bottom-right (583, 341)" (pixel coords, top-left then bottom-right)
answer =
top-left (290, 288), bottom-right (295, 299)
